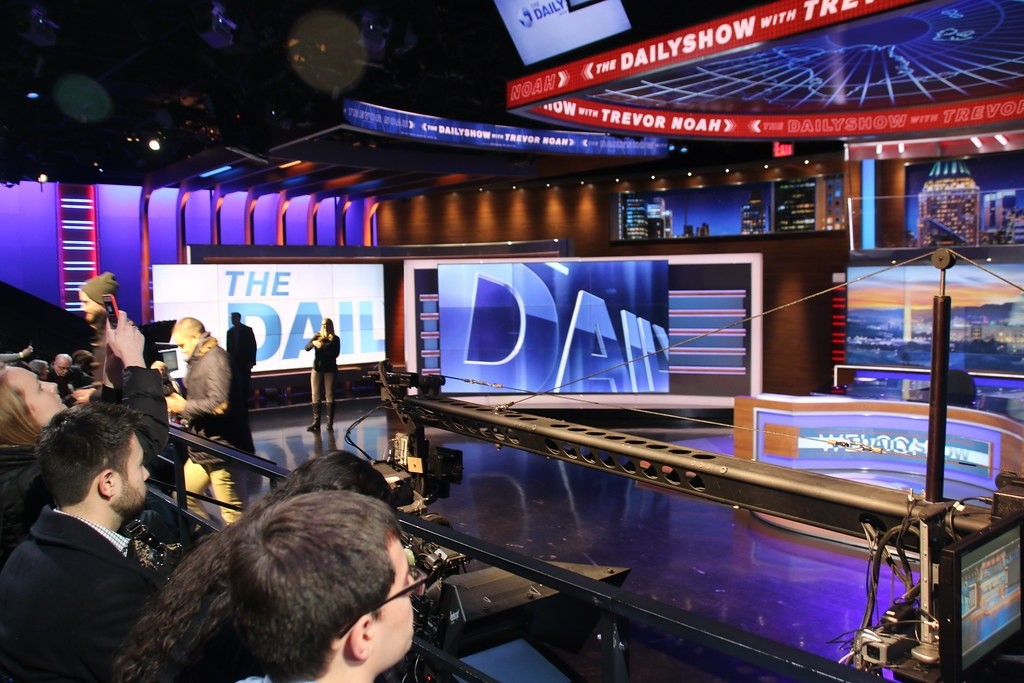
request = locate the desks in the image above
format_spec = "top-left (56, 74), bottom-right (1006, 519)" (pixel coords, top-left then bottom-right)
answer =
top-left (733, 393), bottom-right (1024, 492)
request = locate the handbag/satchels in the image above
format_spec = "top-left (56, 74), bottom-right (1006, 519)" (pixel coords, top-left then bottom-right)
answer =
top-left (126, 519), bottom-right (184, 592)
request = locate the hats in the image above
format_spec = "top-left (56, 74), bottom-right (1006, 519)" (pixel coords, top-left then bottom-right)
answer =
top-left (78, 272), bottom-right (120, 307)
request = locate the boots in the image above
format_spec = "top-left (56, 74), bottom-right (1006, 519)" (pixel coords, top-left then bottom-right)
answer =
top-left (326, 401), bottom-right (337, 432)
top-left (307, 403), bottom-right (323, 433)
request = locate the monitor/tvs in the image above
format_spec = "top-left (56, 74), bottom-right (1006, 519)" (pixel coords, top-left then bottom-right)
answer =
top-left (938, 510), bottom-right (1023, 683)
top-left (491, 0), bottom-right (633, 68)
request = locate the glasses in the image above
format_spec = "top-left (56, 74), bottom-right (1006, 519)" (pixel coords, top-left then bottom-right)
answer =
top-left (338, 567), bottom-right (428, 638)
top-left (57, 364), bottom-right (71, 370)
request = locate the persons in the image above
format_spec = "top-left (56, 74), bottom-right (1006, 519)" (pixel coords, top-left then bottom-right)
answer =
top-left (305, 318), bottom-right (340, 432)
top-left (226, 313), bottom-right (256, 410)
top-left (0, 271), bottom-right (416, 683)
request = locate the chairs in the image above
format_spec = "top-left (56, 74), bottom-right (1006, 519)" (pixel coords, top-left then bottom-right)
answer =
top-left (945, 370), bottom-right (976, 407)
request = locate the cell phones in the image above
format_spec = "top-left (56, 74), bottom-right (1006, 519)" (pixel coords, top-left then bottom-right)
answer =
top-left (102, 294), bottom-right (120, 328)
